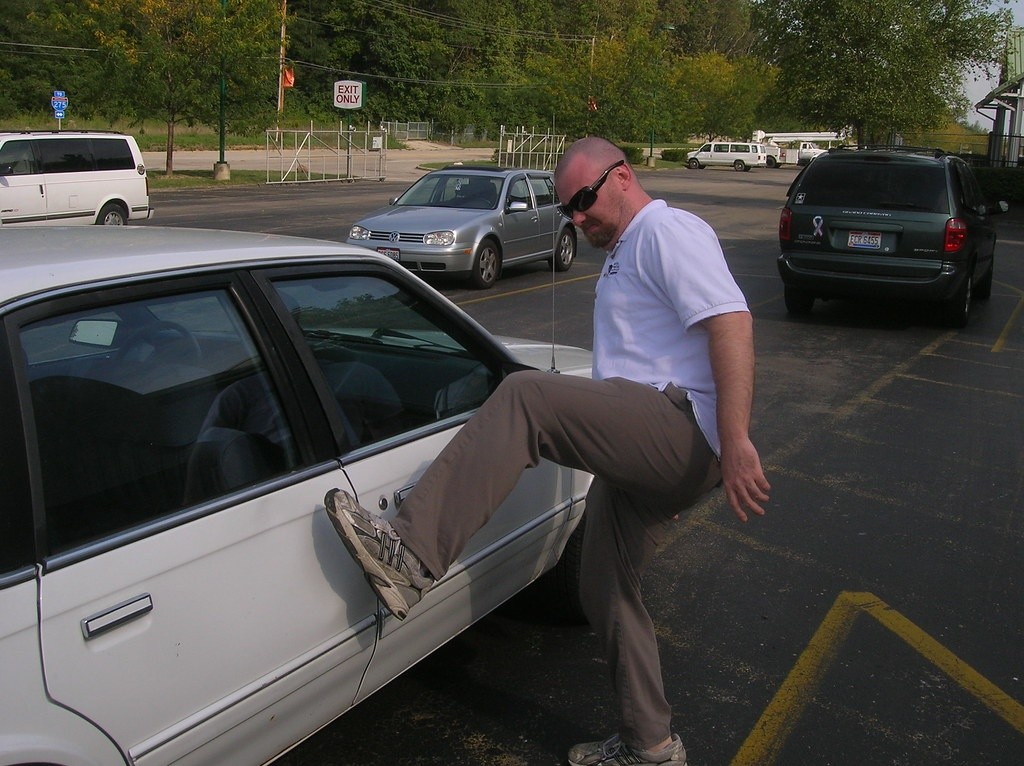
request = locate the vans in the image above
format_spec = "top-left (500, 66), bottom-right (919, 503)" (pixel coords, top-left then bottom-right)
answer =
top-left (686, 142), bottom-right (767, 172)
top-left (0, 129), bottom-right (156, 225)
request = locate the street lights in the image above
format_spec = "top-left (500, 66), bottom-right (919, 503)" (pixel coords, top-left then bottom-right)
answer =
top-left (646, 23), bottom-right (675, 168)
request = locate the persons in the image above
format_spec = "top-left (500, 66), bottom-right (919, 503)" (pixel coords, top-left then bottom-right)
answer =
top-left (186, 364), bottom-right (405, 509)
top-left (323, 137), bottom-right (771, 766)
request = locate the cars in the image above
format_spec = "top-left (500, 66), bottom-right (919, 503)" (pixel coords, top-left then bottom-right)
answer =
top-left (346, 166), bottom-right (577, 290)
top-left (0, 225), bottom-right (596, 766)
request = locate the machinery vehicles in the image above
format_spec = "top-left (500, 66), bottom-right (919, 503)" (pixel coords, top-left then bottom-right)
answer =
top-left (752, 129), bottom-right (838, 168)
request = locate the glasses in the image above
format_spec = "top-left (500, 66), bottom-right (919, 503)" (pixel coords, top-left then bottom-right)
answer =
top-left (558, 159), bottom-right (625, 219)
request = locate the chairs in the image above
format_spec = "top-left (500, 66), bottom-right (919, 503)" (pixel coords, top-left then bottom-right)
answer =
top-left (42, 359), bottom-right (218, 447)
top-left (473, 181), bottom-right (497, 206)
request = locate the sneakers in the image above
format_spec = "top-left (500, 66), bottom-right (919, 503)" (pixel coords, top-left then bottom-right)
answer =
top-left (324, 488), bottom-right (436, 622)
top-left (568, 733), bottom-right (687, 766)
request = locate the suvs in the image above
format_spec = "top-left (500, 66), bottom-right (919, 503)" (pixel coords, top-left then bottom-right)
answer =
top-left (777, 144), bottom-right (1008, 329)
